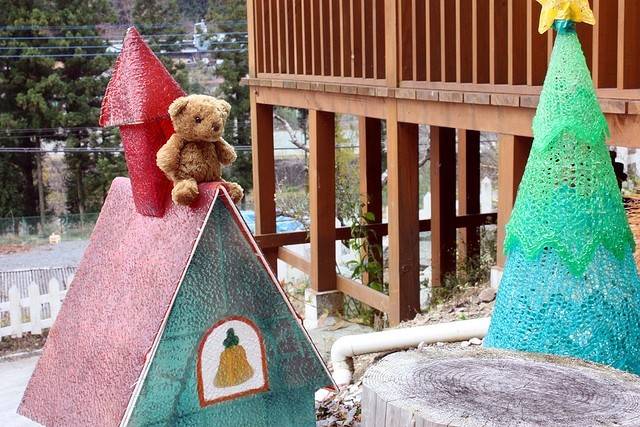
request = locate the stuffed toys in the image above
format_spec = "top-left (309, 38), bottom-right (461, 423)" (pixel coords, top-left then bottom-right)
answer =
top-left (156, 94), bottom-right (245, 206)
